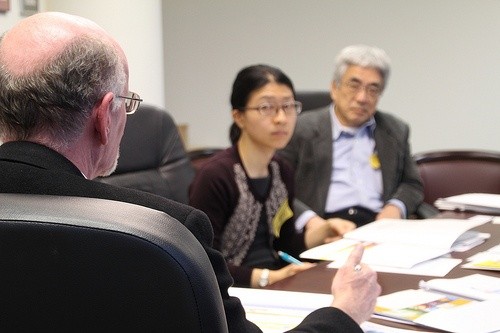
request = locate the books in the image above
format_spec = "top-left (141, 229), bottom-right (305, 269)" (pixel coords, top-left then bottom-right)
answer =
top-left (300, 216), bottom-right (499, 333)
top-left (433, 191), bottom-right (500, 217)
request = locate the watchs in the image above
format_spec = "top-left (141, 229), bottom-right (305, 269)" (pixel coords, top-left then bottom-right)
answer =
top-left (258, 268), bottom-right (269, 287)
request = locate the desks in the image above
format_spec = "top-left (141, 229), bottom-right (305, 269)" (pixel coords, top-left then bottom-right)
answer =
top-left (255, 211), bottom-right (500, 333)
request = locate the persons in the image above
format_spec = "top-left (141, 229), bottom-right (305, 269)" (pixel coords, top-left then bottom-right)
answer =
top-left (187, 63), bottom-right (357, 289)
top-left (101, 102), bottom-right (188, 178)
top-left (0, 11), bottom-right (382, 333)
top-left (273, 44), bottom-right (426, 235)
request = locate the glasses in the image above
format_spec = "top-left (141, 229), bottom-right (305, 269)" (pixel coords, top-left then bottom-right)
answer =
top-left (246, 101), bottom-right (302, 117)
top-left (116, 92), bottom-right (142, 115)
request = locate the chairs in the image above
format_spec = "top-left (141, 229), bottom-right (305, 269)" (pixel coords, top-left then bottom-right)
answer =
top-left (0, 193), bottom-right (228, 333)
top-left (412, 150), bottom-right (500, 207)
top-left (96, 106), bottom-right (196, 205)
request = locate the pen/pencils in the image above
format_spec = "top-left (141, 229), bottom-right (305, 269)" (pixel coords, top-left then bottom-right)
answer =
top-left (355, 265), bottom-right (361, 271)
top-left (278, 251), bottom-right (302, 265)
